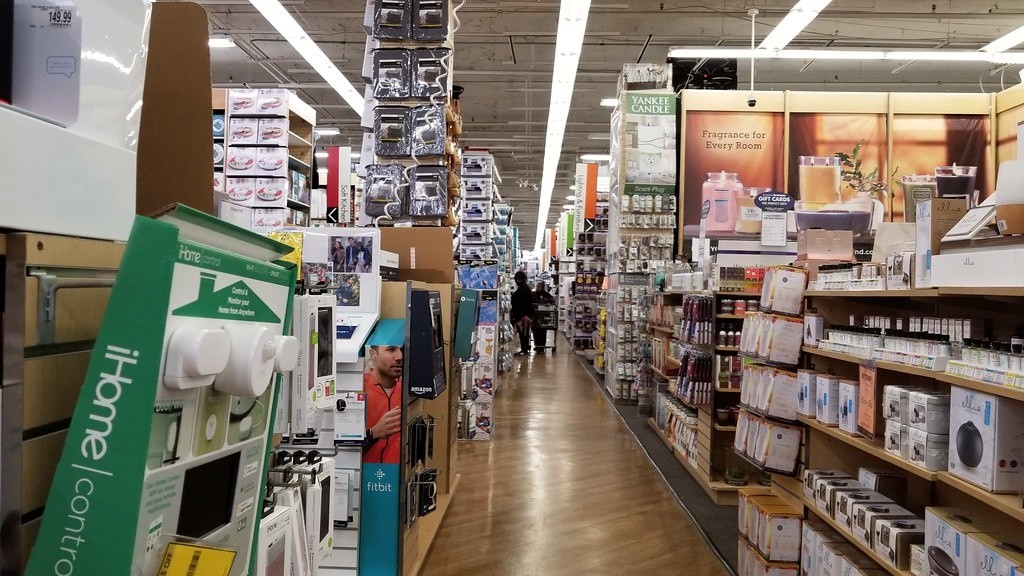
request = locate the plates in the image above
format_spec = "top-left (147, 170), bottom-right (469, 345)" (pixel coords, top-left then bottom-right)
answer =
top-left (212, 97), bottom-right (284, 230)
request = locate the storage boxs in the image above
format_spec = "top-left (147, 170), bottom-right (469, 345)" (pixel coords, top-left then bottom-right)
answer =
top-left (716, 195), bottom-right (1024, 576)
top-left (22, 200), bottom-right (299, 576)
top-left (251, 292), bottom-right (338, 576)
top-left (454, 153), bottom-right (500, 441)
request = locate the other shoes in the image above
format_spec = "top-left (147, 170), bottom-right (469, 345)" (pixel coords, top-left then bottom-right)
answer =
top-left (534, 347), bottom-right (544, 352)
top-left (514, 349), bottom-right (528, 356)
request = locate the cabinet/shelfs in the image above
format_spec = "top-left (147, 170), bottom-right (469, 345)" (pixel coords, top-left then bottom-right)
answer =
top-left (287, 90), bottom-right (317, 228)
top-left (737, 287), bottom-right (1024, 576)
top-left (646, 290), bottom-right (771, 506)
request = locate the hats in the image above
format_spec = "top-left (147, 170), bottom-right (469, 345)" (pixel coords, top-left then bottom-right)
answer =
top-left (513, 271), bottom-right (527, 280)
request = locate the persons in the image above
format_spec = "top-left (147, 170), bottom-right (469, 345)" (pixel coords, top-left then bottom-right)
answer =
top-left (363, 345), bottom-right (401, 464)
top-left (302, 236), bottom-right (372, 285)
top-left (335, 274), bottom-right (355, 306)
top-left (510, 271), bottom-right (534, 356)
top-left (531, 282), bottom-right (556, 352)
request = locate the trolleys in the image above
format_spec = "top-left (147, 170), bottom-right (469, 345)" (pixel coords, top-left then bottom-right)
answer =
top-left (532, 303), bottom-right (560, 354)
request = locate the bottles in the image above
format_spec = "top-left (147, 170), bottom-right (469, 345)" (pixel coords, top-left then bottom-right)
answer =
top-left (702, 171), bottom-right (770, 234)
top-left (716, 298), bottom-right (760, 348)
top-left (797, 155), bottom-right (842, 209)
top-left (716, 405), bottom-right (739, 427)
top-left (901, 165), bottom-right (978, 224)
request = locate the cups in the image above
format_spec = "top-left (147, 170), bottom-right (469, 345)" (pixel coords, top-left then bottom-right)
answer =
top-left (724, 443), bottom-right (750, 486)
top-left (756, 468), bottom-right (771, 487)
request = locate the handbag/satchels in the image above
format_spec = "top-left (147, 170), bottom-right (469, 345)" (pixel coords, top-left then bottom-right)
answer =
top-left (516, 315), bottom-right (533, 336)
